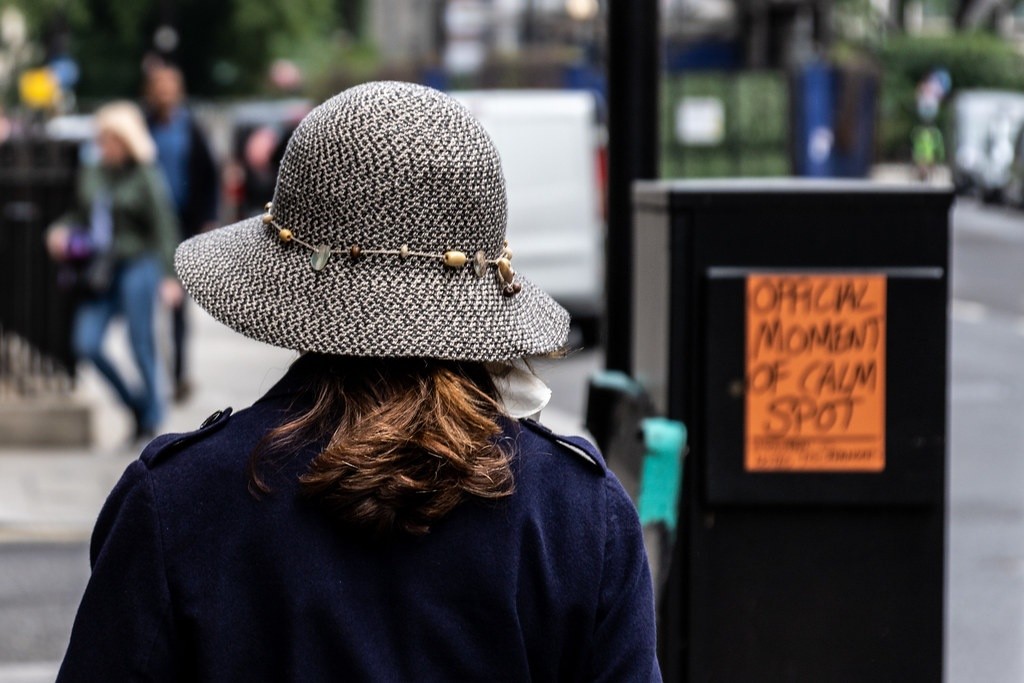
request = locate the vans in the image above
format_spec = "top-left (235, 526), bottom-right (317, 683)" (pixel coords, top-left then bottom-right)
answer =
top-left (447, 89), bottom-right (609, 348)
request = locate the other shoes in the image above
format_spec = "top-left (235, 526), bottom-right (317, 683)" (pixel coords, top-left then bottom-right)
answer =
top-left (176, 380), bottom-right (194, 404)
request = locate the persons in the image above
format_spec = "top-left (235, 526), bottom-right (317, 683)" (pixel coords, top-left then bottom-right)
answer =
top-left (47, 55), bottom-right (224, 447)
top-left (910, 114), bottom-right (944, 182)
top-left (560, 33), bottom-right (609, 216)
top-left (785, 16), bottom-right (882, 179)
top-left (231, 59), bottom-right (311, 223)
top-left (55, 81), bottom-right (661, 683)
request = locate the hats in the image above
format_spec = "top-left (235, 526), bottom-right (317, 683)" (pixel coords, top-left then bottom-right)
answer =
top-left (176, 80), bottom-right (570, 358)
